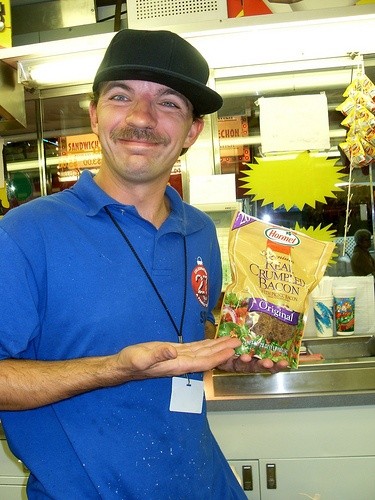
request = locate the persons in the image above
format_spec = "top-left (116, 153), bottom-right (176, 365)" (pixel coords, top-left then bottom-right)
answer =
top-left (0, 27), bottom-right (295, 500)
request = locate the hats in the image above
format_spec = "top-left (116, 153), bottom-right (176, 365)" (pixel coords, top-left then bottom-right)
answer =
top-left (92, 29), bottom-right (224, 114)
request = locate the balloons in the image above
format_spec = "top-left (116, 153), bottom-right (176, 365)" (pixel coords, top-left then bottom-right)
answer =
top-left (7, 171), bottom-right (32, 203)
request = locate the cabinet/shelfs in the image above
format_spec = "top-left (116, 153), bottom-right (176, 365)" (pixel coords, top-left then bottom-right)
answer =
top-left (0, 420), bottom-right (31, 500)
top-left (206, 405), bottom-right (375, 500)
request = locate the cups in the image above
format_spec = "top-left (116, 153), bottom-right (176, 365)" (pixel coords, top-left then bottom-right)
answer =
top-left (332, 287), bottom-right (357, 336)
top-left (312, 295), bottom-right (334, 338)
